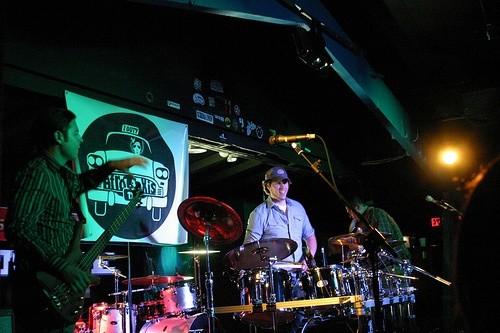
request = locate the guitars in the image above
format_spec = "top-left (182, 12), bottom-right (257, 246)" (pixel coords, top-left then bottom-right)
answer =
top-left (35, 180), bottom-right (144, 325)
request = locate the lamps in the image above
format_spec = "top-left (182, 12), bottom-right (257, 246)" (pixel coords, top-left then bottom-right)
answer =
top-left (293, 19), bottom-right (335, 70)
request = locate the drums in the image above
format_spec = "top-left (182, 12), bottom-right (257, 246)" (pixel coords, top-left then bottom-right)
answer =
top-left (159, 282), bottom-right (199, 315)
top-left (291, 264), bottom-right (415, 333)
top-left (238, 261), bottom-right (297, 327)
top-left (139, 300), bottom-right (165, 321)
top-left (139, 312), bottom-right (220, 333)
top-left (92, 302), bottom-right (138, 333)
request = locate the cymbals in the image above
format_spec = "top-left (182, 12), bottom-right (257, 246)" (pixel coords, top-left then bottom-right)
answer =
top-left (97, 255), bottom-right (128, 259)
top-left (122, 275), bottom-right (195, 285)
top-left (224, 238), bottom-right (297, 271)
top-left (327, 232), bottom-right (392, 245)
top-left (340, 240), bottom-right (407, 263)
top-left (178, 249), bottom-right (220, 256)
top-left (178, 196), bottom-right (243, 245)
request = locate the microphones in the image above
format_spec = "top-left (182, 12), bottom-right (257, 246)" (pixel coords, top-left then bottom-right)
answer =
top-left (269, 134), bottom-right (319, 145)
top-left (302, 239), bottom-right (317, 268)
top-left (425, 196), bottom-right (454, 212)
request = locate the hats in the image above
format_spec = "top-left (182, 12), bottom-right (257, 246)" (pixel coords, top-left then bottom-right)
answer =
top-left (265, 167), bottom-right (292, 184)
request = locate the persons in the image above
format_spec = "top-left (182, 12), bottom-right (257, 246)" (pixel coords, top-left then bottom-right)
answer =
top-left (346, 200), bottom-right (411, 326)
top-left (243, 167), bottom-right (317, 302)
top-left (4, 106), bottom-right (149, 333)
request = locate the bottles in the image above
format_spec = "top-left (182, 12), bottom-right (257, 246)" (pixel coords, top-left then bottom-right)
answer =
top-left (74, 319), bottom-right (86, 333)
